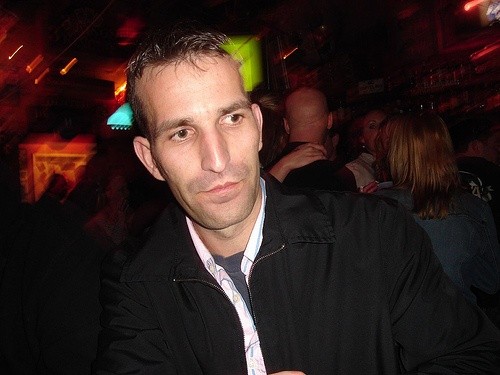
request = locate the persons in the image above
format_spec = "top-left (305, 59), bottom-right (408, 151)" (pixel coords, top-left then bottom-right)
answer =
top-left (97, 26), bottom-right (500, 375)
top-left (0, 67), bottom-right (499, 375)
